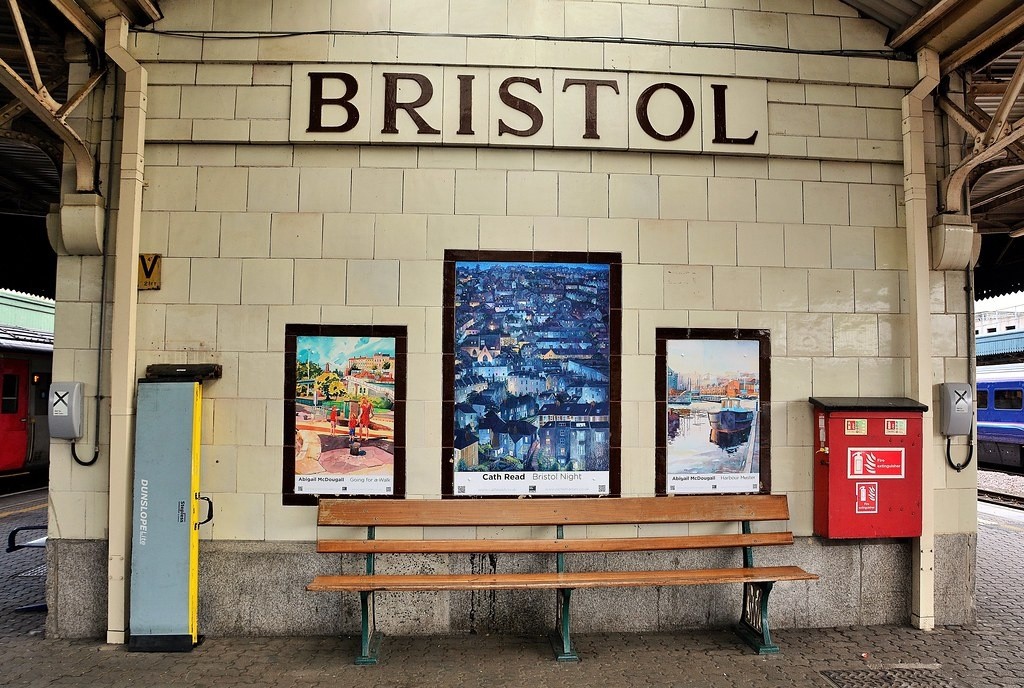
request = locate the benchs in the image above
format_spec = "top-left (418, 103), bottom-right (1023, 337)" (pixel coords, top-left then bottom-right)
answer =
top-left (304, 495), bottom-right (822, 665)
top-left (6, 525), bottom-right (48, 613)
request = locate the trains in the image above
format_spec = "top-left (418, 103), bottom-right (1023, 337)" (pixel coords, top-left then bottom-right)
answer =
top-left (977, 362), bottom-right (1024, 448)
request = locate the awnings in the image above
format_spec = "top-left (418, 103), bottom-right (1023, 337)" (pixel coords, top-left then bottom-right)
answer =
top-left (0, 326), bottom-right (56, 353)
